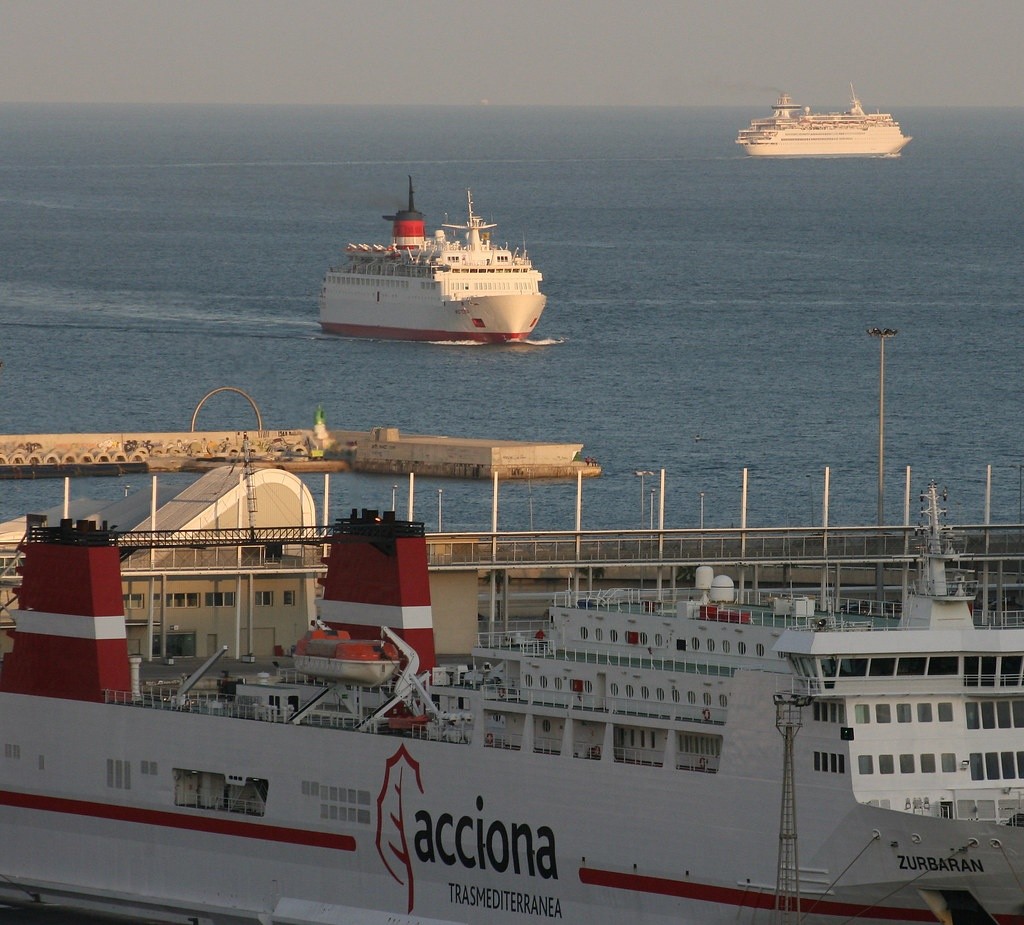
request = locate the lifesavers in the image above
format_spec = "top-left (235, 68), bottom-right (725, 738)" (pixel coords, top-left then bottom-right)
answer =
top-left (593, 746), bottom-right (601, 756)
top-left (499, 687), bottom-right (505, 698)
top-left (486, 733), bottom-right (494, 745)
top-left (699, 757), bottom-right (705, 769)
top-left (703, 709), bottom-right (710, 721)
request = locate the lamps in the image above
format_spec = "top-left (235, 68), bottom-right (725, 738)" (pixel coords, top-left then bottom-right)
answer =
top-left (962, 760), bottom-right (969, 769)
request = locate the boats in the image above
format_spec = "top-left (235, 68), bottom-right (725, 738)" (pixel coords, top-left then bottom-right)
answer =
top-left (1, 334), bottom-right (1023, 925)
top-left (315, 173), bottom-right (547, 344)
top-left (733, 81), bottom-right (914, 156)
